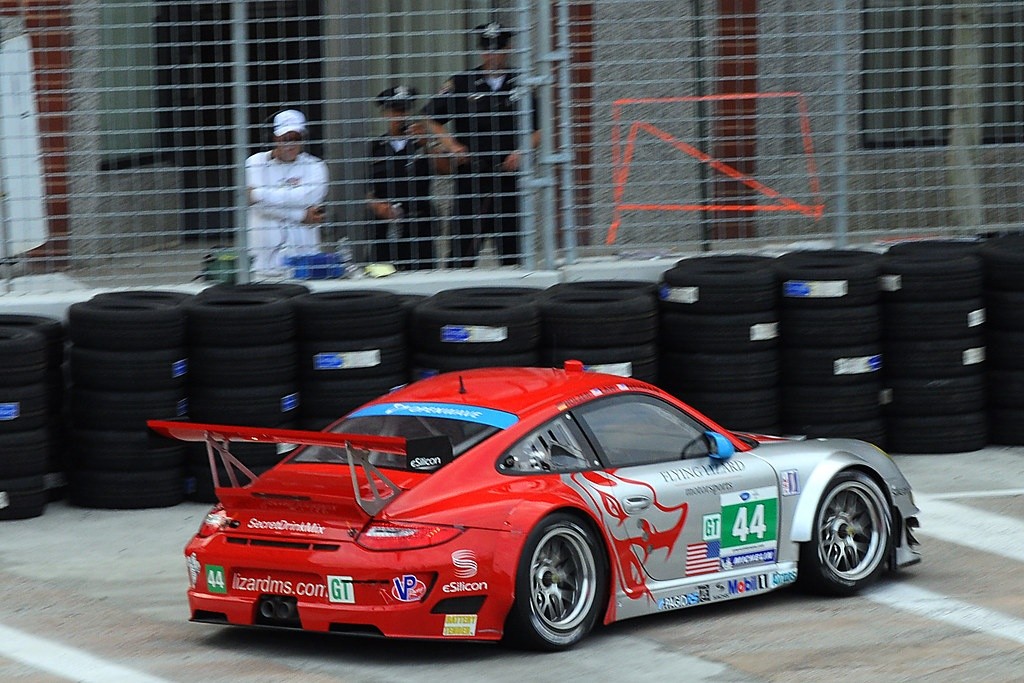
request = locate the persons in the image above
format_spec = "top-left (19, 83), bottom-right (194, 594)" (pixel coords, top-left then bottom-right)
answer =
top-left (415, 21), bottom-right (546, 269)
top-left (363, 85), bottom-right (437, 271)
top-left (242, 110), bottom-right (332, 279)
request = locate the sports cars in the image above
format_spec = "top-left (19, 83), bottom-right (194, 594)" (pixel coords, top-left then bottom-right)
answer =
top-left (150, 358), bottom-right (923, 658)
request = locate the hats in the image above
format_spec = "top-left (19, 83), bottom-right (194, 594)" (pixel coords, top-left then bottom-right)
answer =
top-left (474, 21), bottom-right (510, 50)
top-left (371, 86), bottom-right (418, 112)
top-left (273, 110), bottom-right (308, 137)
